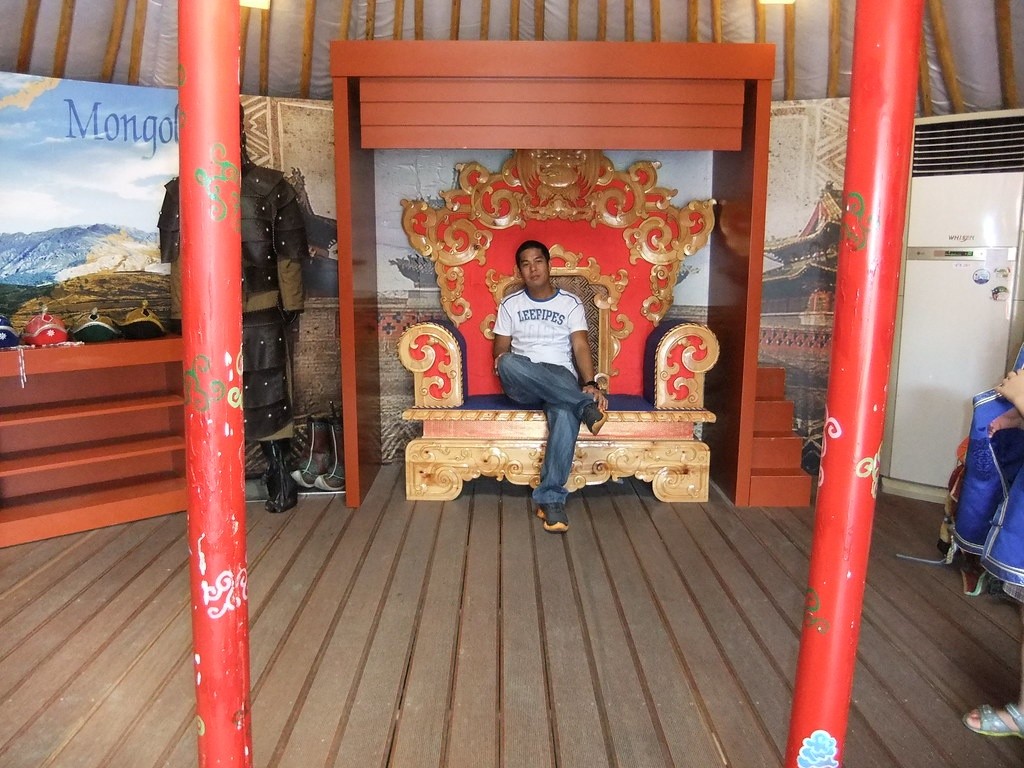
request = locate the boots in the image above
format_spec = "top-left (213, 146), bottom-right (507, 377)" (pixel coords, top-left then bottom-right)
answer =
top-left (290, 417), bottom-right (345, 491)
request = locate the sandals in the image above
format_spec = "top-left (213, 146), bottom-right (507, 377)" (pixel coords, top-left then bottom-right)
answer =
top-left (963, 700), bottom-right (1024, 737)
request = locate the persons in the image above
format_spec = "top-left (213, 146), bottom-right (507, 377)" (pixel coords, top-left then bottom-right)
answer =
top-left (963, 367), bottom-right (1023, 741)
top-left (158, 101), bottom-right (304, 513)
top-left (490, 241), bottom-right (609, 533)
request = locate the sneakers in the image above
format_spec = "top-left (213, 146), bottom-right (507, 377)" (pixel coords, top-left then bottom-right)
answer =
top-left (581, 397), bottom-right (608, 437)
top-left (536, 498), bottom-right (570, 533)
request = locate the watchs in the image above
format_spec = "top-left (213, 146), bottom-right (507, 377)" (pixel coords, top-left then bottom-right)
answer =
top-left (584, 380), bottom-right (601, 390)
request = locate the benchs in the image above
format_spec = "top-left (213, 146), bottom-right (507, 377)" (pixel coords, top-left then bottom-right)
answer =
top-left (395, 149), bottom-right (719, 501)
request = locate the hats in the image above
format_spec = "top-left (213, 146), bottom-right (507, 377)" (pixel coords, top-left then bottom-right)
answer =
top-left (1, 300), bottom-right (164, 349)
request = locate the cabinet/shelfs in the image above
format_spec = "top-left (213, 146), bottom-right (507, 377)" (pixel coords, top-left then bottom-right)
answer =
top-left (0, 337), bottom-right (187, 547)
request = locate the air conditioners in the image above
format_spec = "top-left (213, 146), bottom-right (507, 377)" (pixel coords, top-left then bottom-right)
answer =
top-left (878, 108), bottom-right (1024, 506)
